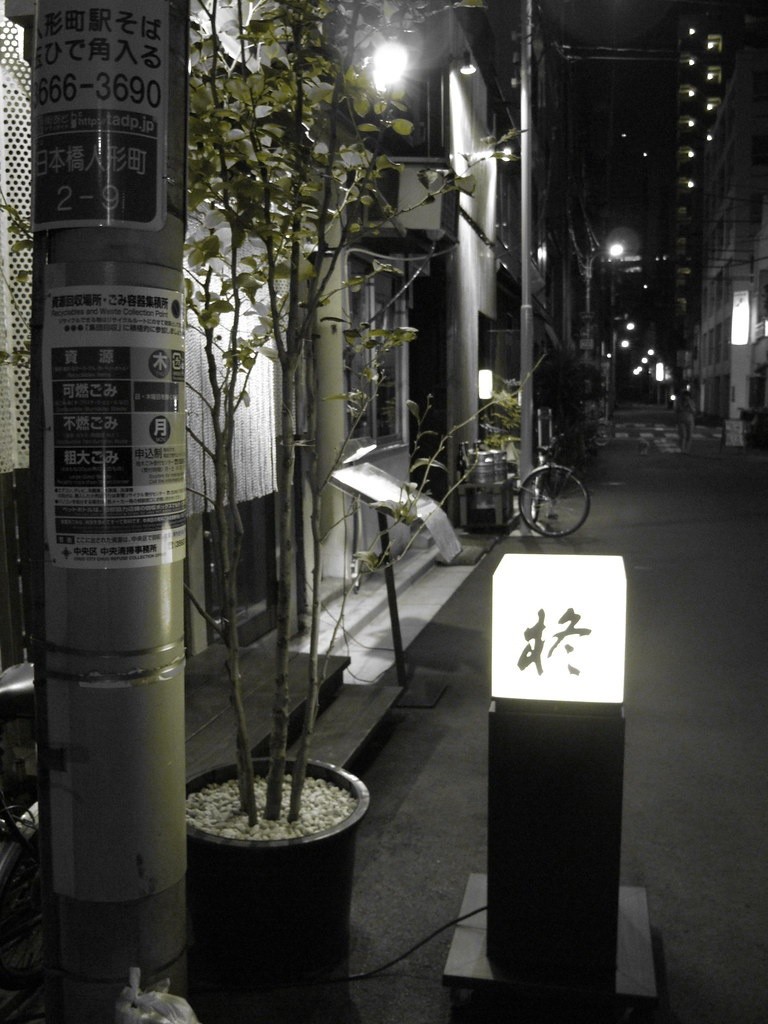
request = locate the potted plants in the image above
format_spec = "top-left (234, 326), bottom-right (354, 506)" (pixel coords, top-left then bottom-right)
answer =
top-left (158, 0), bottom-right (527, 1007)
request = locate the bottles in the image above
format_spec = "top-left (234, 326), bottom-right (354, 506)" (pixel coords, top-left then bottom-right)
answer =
top-left (455, 439), bottom-right (484, 483)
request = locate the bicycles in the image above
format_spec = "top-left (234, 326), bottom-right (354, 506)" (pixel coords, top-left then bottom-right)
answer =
top-left (589, 410), bottom-right (613, 448)
top-left (518, 434), bottom-right (590, 537)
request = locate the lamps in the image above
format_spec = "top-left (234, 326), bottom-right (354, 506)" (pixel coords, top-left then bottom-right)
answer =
top-left (448, 51), bottom-right (476, 75)
top-left (491, 552), bottom-right (630, 708)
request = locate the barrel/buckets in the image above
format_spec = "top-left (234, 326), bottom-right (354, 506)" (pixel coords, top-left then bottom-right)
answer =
top-left (466, 451), bottom-right (494, 484)
top-left (489, 450), bottom-right (507, 481)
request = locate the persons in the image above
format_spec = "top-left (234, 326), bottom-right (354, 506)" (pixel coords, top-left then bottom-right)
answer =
top-left (675, 391), bottom-right (696, 455)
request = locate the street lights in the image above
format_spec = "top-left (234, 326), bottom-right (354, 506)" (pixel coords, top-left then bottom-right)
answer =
top-left (584, 243), bottom-right (625, 368)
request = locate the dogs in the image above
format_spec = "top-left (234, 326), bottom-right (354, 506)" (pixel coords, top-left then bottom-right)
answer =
top-left (639, 437), bottom-right (651, 458)
